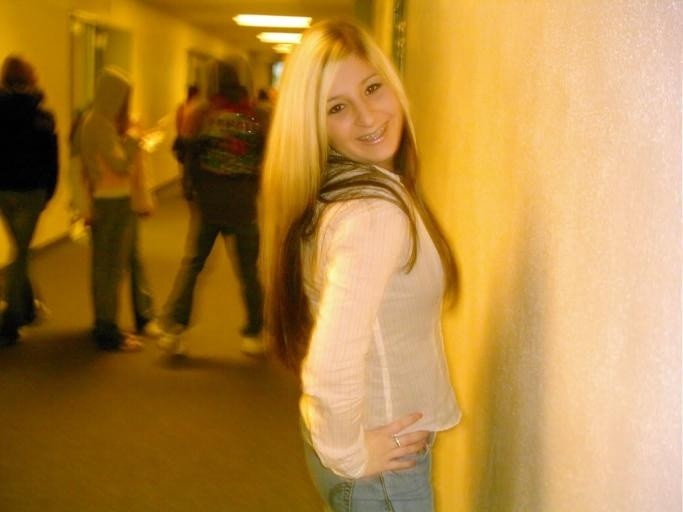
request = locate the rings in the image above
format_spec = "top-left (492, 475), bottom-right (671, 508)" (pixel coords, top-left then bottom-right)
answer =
top-left (394, 437), bottom-right (401, 448)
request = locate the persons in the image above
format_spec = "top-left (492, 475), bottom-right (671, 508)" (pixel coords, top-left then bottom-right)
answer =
top-left (257, 16), bottom-right (463, 512)
top-left (0, 56), bottom-right (60, 347)
top-left (121, 122), bottom-right (160, 340)
top-left (152, 53), bottom-right (272, 360)
top-left (72, 65), bottom-right (146, 353)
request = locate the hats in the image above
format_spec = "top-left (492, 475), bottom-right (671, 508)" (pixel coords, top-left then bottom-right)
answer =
top-left (1, 56), bottom-right (36, 86)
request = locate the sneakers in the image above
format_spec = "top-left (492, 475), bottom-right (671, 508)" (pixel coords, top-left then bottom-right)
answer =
top-left (117, 334), bottom-right (144, 353)
top-left (240, 334), bottom-right (264, 355)
top-left (139, 317), bottom-right (163, 335)
top-left (154, 329), bottom-right (178, 351)
